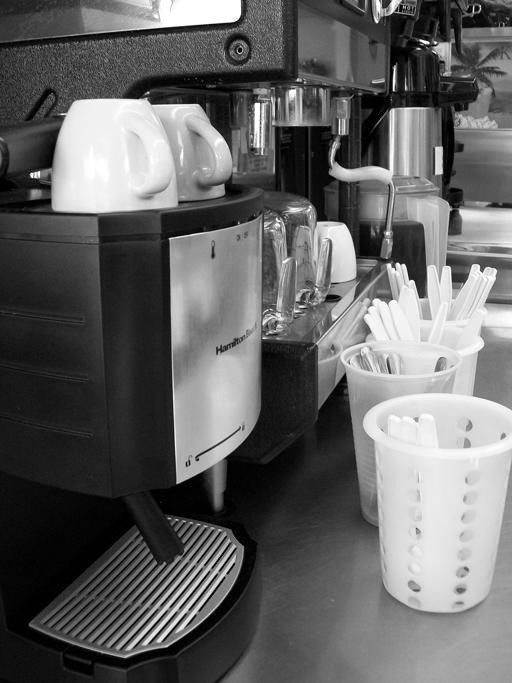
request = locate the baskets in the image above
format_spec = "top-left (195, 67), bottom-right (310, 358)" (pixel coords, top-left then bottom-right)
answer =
top-left (363, 390), bottom-right (512, 619)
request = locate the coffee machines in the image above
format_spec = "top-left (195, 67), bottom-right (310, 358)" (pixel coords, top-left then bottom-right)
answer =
top-left (0, 0), bottom-right (296, 566)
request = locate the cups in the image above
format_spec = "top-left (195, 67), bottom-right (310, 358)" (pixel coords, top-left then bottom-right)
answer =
top-left (52, 98), bottom-right (233, 214)
top-left (340, 293), bottom-right (489, 530)
top-left (262, 189), bottom-right (359, 335)
